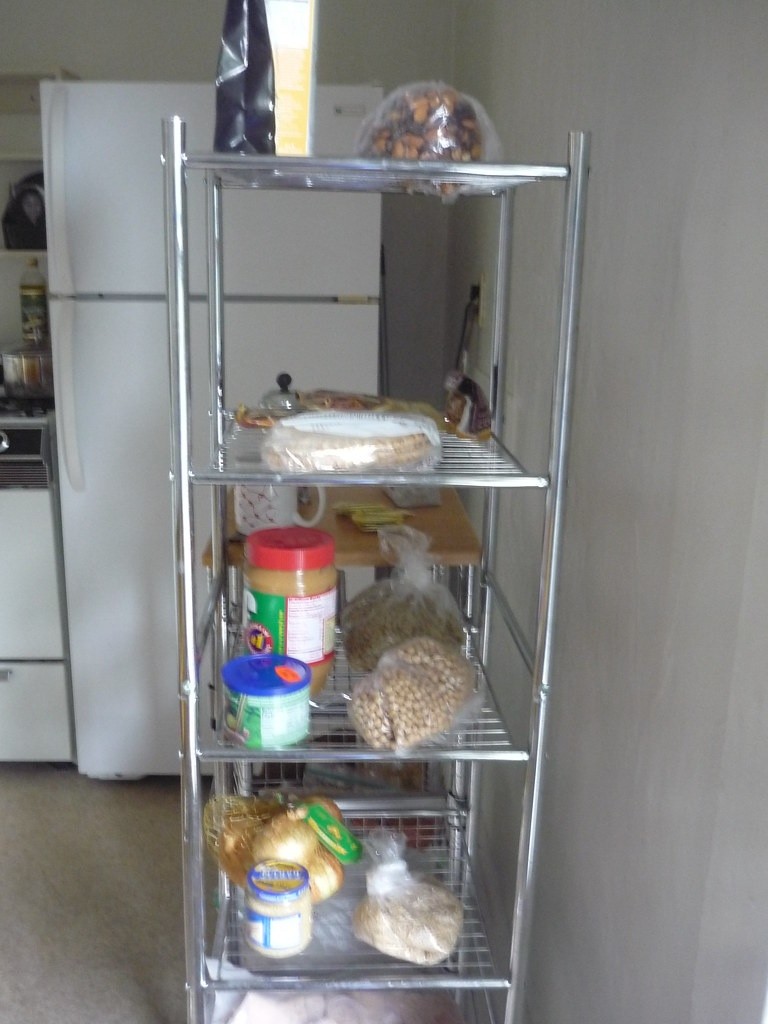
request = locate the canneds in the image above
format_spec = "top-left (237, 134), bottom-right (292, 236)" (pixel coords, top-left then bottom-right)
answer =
top-left (241, 859), bottom-right (312, 961)
top-left (220, 652), bottom-right (310, 750)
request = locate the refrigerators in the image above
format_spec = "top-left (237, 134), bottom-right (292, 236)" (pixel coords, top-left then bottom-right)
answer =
top-left (35, 76), bottom-right (390, 794)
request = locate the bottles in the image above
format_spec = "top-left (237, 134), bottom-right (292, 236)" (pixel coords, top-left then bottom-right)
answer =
top-left (19, 256), bottom-right (45, 342)
top-left (243, 530), bottom-right (343, 697)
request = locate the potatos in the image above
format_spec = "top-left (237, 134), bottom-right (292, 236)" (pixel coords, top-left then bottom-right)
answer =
top-left (249, 796), bottom-right (345, 904)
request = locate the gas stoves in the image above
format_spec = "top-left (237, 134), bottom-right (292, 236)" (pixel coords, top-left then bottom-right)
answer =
top-left (0, 390), bottom-right (59, 488)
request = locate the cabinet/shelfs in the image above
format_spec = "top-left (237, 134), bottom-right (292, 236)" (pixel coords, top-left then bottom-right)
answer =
top-left (153, 106), bottom-right (592, 1024)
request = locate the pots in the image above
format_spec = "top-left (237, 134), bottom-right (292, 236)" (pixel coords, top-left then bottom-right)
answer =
top-left (2, 344), bottom-right (51, 407)
